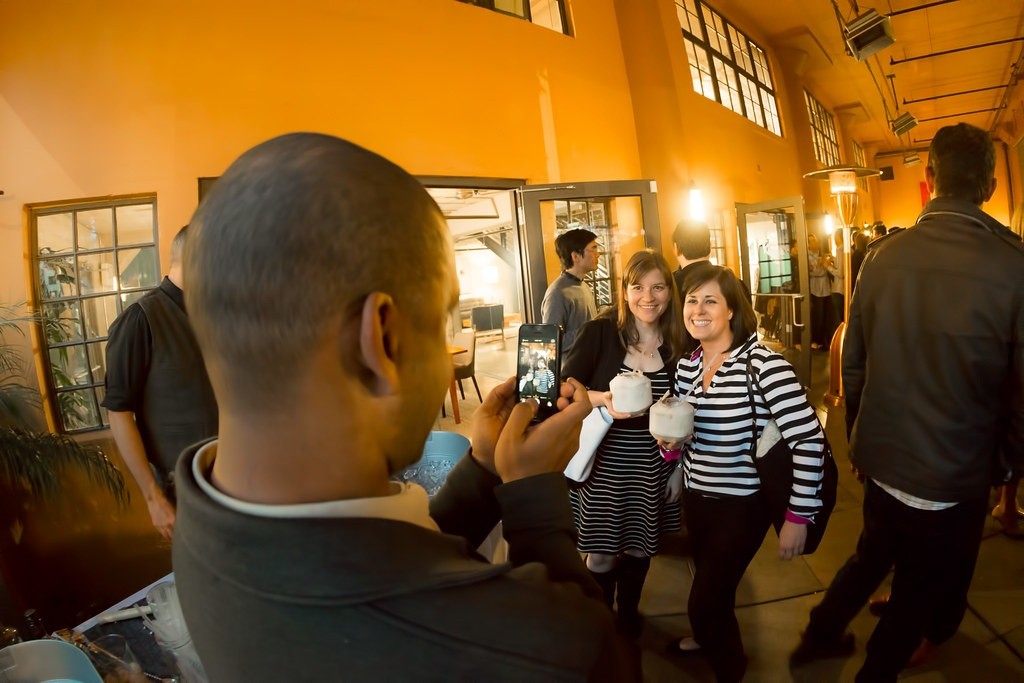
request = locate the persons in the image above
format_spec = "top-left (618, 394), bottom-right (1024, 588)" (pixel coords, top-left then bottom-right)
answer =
top-left (561, 250), bottom-right (827, 683)
top-left (98, 225), bottom-right (218, 541)
top-left (798, 122), bottom-right (1024, 683)
top-left (541, 228), bottom-right (600, 371)
top-left (519, 355), bottom-right (554, 396)
top-left (669, 215), bottom-right (752, 315)
top-left (167, 131), bottom-right (644, 683)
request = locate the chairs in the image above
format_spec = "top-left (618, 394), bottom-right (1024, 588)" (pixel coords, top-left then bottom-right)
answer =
top-left (440, 331), bottom-right (485, 419)
top-left (470, 303), bottom-right (506, 341)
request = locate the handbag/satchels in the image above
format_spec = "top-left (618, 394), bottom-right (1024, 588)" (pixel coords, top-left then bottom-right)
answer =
top-left (548, 385), bottom-right (555, 397)
top-left (745, 345), bottom-right (838, 554)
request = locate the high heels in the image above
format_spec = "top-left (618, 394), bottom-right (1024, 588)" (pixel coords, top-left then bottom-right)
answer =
top-left (990, 505), bottom-right (1024, 538)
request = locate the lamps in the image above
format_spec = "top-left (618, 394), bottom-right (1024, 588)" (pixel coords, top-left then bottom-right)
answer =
top-left (822, 208), bottom-right (838, 239)
top-left (903, 154), bottom-right (923, 168)
top-left (686, 180), bottom-right (705, 219)
top-left (803, 162), bottom-right (883, 196)
top-left (843, 6), bottom-right (897, 62)
top-left (892, 111), bottom-right (919, 137)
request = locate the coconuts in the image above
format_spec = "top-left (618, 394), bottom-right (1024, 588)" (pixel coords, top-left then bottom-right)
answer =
top-left (609, 368), bottom-right (653, 415)
top-left (526, 374), bottom-right (540, 386)
top-left (649, 396), bottom-right (694, 442)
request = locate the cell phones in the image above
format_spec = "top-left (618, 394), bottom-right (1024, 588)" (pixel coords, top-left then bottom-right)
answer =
top-left (517, 323), bottom-right (563, 423)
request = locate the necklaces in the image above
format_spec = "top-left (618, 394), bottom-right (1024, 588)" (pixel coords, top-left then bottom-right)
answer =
top-left (630, 334), bottom-right (660, 358)
top-left (701, 353), bottom-right (733, 372)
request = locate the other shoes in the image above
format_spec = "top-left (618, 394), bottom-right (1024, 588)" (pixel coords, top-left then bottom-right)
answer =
top-left (908, 637), bottom-right (958, 674)
top-left (666, 636), bottom-right (701, 656)
top-left (718, 656), bottom-right (747, 683)
top-left (869, 593), bottom-right (891, 611)
top-left (853, 657), bottom-right (898, 683)
top-left (790, 632), bottom-right (855, 668)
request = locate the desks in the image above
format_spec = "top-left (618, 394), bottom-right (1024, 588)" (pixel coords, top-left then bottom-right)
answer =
top-left (446, 344), bottom-right (469, 426)
